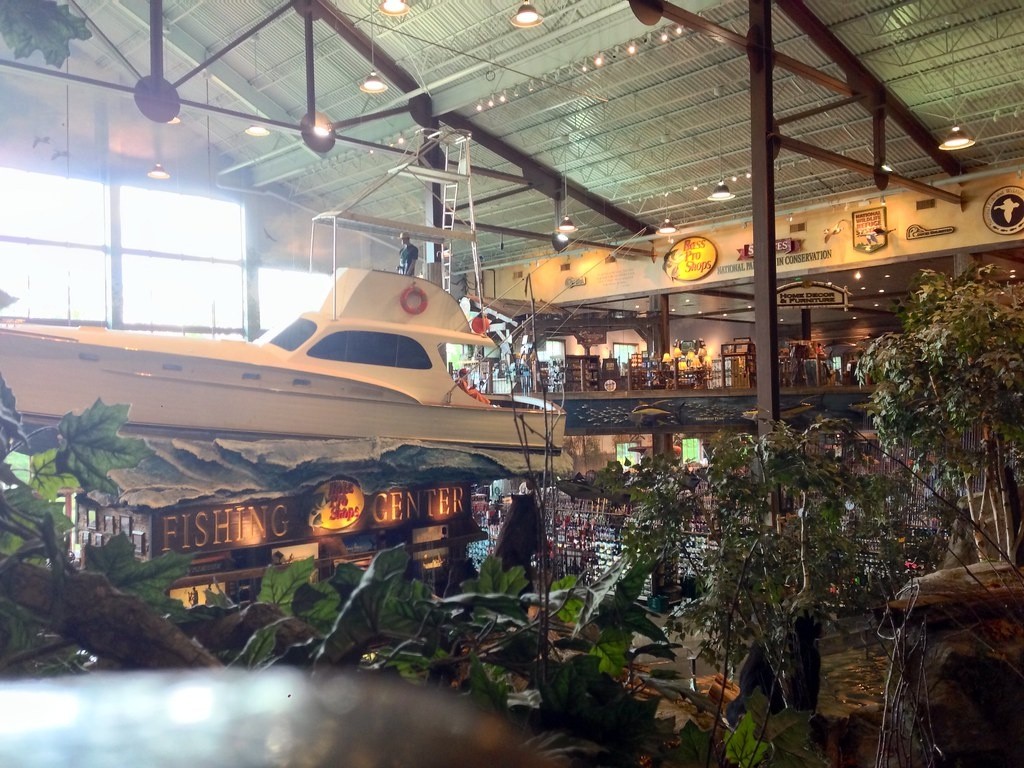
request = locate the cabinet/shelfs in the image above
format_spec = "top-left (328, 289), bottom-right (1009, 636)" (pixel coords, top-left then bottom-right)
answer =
top-left (469, 505), bottom-right (946, 611)
top-left (565, 337), bottom-right (808, 392)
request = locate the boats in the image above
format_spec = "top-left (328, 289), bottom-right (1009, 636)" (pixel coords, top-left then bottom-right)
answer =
top-left (0, 124), bottom-right (568, 456)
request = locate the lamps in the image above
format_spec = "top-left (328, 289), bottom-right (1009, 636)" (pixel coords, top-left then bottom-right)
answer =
top-left (707, 86), bottom-right (736, 200)
top-left (148, 164), bottom-right (170, 179)
top-left (368, 132), bottom-right (406, 153)
top-left (360, 0), bottom-right (388, 94)
top-left (882, 162), bottom-right (895, 172)
top-left (660, 133), bottom-right (676, 232)
top-left (245, 33), bottom-right (270, 138)
top-left (380, 0), bottom-right (410, 16)
top-left (558, 134), bottom-right (579, 241)
top-left (1017, 166), bottom-right (1023, 180)
top-left (880, 196), bottom-right (886, 205)
top-left (938, 15), bottom-right (1024, 150)
top-left (511, 0), bottom-right (543, 27)
